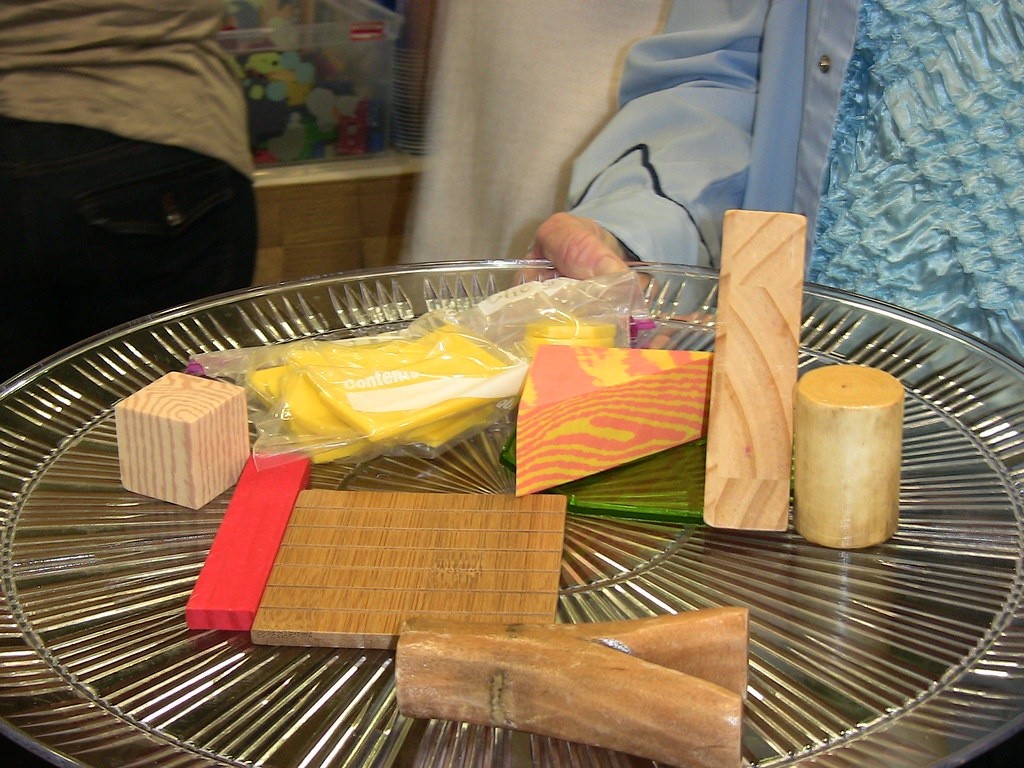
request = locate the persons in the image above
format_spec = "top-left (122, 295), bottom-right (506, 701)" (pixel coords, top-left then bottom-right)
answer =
top-left (514, 0), bottom-right (1024, 364)
top-left (0, 0), bottom-right (259, 385)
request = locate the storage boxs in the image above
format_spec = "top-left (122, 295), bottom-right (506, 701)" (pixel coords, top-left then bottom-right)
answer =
top-left (212, 0), bottom-right (404, 168)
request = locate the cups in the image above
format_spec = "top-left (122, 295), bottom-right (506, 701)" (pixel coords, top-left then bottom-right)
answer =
top-left (385, 0), bottom-right (436, 152)
